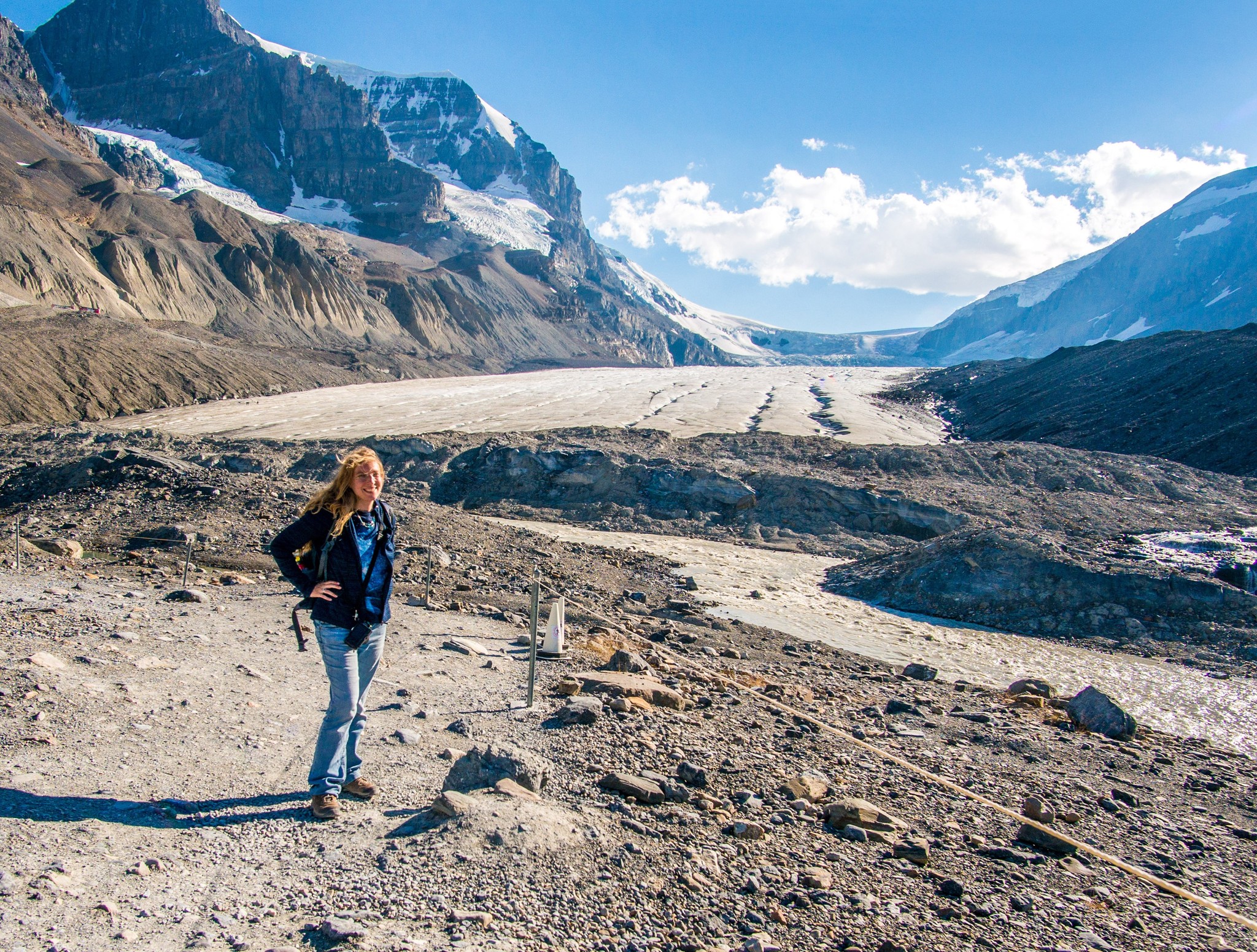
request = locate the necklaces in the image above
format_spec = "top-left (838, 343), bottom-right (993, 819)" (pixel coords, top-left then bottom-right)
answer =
top-left (356, 511), bottom-right (376, 528)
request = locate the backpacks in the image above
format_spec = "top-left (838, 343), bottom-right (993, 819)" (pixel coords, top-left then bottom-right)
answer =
top-left (292, 500), bottom-right (396, 586)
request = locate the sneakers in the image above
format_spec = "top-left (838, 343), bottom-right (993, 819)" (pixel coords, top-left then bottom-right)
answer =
top-left (311, 794), bottom-right (341, 817)
top-left (343, 777), bottom-right (378, 797)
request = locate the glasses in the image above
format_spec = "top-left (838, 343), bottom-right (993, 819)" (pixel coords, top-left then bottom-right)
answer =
top-left (353, 472), bottom-right (381, 480)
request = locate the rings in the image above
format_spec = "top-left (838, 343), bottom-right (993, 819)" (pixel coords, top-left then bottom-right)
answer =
top-left (323, 589), bottom-right (326, 592)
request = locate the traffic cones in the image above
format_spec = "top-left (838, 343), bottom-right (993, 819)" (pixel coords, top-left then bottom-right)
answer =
top-left (535, 597), bottom-right (571, 659)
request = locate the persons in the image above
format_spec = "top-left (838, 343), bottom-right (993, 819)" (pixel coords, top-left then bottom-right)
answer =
top-left (271, 447), bottom-right (395, 818)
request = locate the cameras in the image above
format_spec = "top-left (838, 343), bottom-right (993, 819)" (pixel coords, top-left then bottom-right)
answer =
top-left (344, 622), bottom-right (373, 650)
